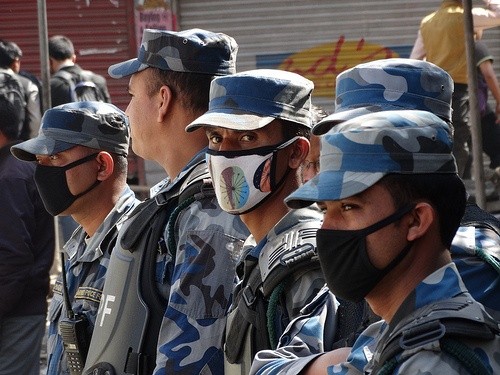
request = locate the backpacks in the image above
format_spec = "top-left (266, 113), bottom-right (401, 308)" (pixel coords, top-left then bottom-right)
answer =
top-left (0, 66), bottom-right (25, 140)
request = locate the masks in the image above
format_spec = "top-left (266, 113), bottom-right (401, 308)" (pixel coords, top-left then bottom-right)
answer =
top-left (316, 201), bottom-right (417, 300)
top-left (205, 135), bottom-right (300, 215)
top-left (34, 152), bottom-right (102, 216)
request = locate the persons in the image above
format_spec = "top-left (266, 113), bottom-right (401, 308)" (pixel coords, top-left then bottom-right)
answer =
top-left (406, 0), bottom-right (500, 179)
top-left (0, 68), bottom-right (56, 375)
top-left (283, 110), bottom-right (500, 375)
top-left (0, 41), bottom-right (41, 141)
top-left (462, 28), bottom-right (500, 201)
top-left (248, 59), bottom-right (500, 375)
top-left (10, 102), bottom-right (144, 375)
top-left (82, 29), bottom-right (250, 374)
top-left (182, 70), bottom-right (327, 375)
top-left (48, 35), bottom-right (111, 109)
top-left (301, 104), bottom-right (330, 209)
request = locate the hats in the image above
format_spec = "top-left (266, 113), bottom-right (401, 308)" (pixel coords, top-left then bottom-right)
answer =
top-left (284, 110), bottom-right (458, 209)
top-left (312, 58), bottom-right (454, 136)
top-left (108, 29), bottom-right (239, 79)
top-left (185, 69), bottom-right (314, 132)
top-left (10, 101), bottom-right (129, 162)
